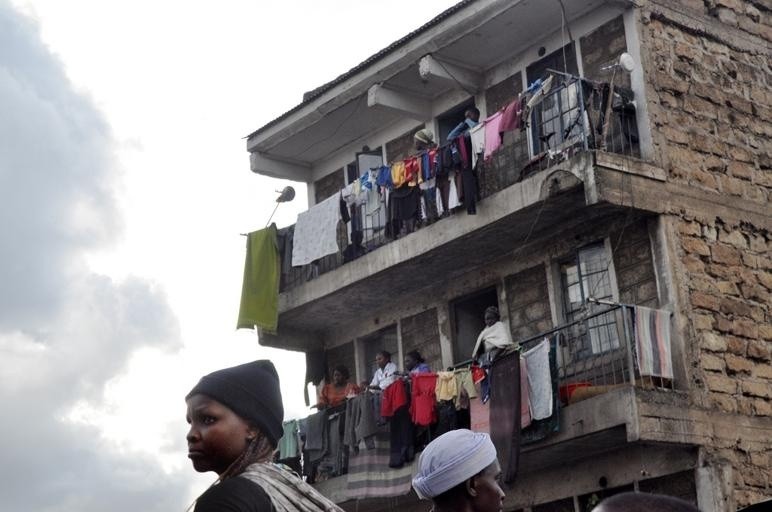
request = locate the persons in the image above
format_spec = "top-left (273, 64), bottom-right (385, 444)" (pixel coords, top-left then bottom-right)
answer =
top-left (413, 128), bottom-right (436, 151)
top-left (185, 359), bottom-right (347, 512)
top-left (472, 306), bottom-right (513, 361)
top-left (591, 490), bottom-right (707, 512)
top-left (411, 428), bottom-right (505, 512)
top-left (447, 106), bottom-right (480, 141)
top-left (369, 350), bottom-right (398, 386)
top-left (405, 351), bottom-right (431, 373)
top-left (318, 365), bottom-right (360, 411)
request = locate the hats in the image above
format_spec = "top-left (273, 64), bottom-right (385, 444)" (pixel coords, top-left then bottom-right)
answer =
top-left (486, 306), bottom-right (500, 316)
top-left (414, 128), bottom-right (434, 144)
top-left (186, 358), bottom-right (284, 447)
top-left (411, 428), bottom-right (498, 503)
